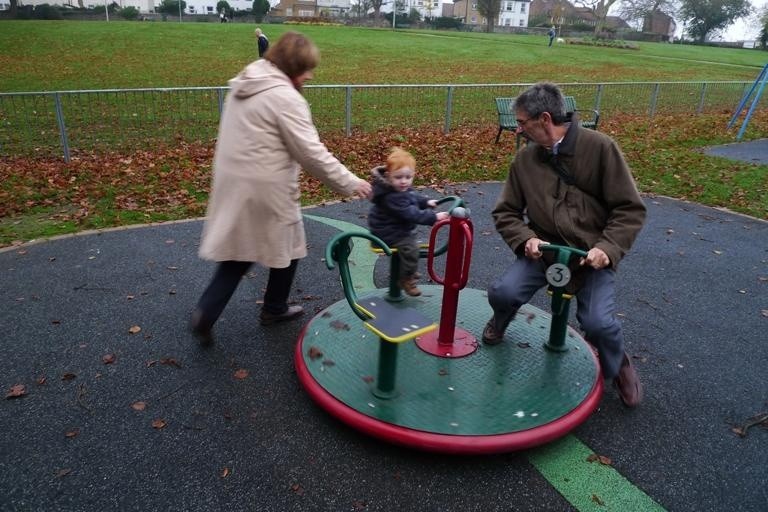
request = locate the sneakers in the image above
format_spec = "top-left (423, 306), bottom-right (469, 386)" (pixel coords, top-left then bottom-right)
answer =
top-left (187, 315), bottom-right (216, 346)
top-left (404, 272), bottom-right (423, 297)
top-left (261, 305), bottom-right (304, 325)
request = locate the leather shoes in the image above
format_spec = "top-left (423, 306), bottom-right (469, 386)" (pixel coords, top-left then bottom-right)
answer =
top-left (612, 352), bottom-right (645, 409)
top-left (482, 314), bottom-right (514, 344)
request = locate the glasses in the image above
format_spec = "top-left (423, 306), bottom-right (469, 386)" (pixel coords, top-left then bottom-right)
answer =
top-left (514, 111), bottom-right (540, 126)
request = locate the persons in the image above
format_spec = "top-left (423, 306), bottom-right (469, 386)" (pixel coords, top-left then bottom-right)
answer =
top-left (548, 25), bottom-right (556, 48)
top-left (481, 81), bottom-right (647, 409)
top-left (187, 31), bottom-right (372, 350)
top-left (254, 27), bottom-right (269, 57)
top-left (367, 146), bottom-right (450, 296)
